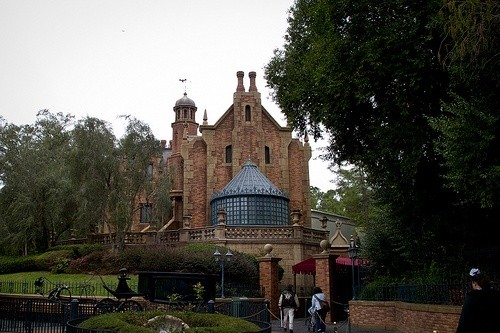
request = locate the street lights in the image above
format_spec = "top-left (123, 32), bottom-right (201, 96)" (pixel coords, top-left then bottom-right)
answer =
top-left (345, 234), bottom-right (360, 301)
top-left (213, 248), bottom-right (233, 297)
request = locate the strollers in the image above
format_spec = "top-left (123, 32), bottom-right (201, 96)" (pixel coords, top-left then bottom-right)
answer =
top-left (305, 308), bottom-right (316, 333)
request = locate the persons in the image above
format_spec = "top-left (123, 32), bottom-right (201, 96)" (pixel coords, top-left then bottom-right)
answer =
top-left (277, 284), bottom-right (300, 333)
top-left (307, 286), bottom-right (330, 333)
top-left (455, 273), bottom-right (500, 333)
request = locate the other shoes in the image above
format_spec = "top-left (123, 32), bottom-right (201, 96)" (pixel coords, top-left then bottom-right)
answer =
top-left (284, 328), bottom-right (287, 332)
top-left (289, 329), bottom-right (293, 333)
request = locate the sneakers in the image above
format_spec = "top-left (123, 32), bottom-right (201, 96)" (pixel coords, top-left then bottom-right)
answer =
top-left (320, 332), bottom-right (324, 333)
top-left (317, 328), bottom-right (322, 332)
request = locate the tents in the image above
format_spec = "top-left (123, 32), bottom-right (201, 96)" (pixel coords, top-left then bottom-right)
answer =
top-left (335, 255), bottom-right (369, 293)
top-left (292, 256), bottom-right (315, 293)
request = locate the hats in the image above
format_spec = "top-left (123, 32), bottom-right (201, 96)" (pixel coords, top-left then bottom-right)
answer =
top-left (286, 284), bottom-right (293, 290)
top-left (469, 268), bottom-right (481, 278)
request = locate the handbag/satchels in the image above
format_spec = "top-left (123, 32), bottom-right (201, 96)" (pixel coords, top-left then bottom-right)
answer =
top-left (319, 300), bottom-right (330, 311)
